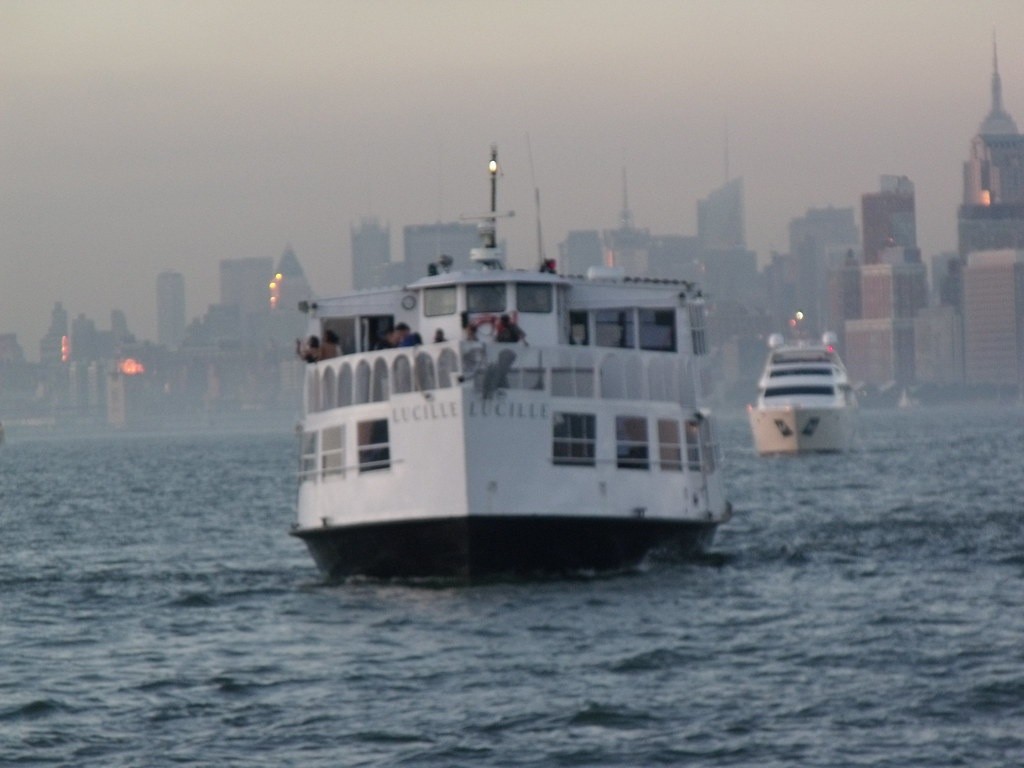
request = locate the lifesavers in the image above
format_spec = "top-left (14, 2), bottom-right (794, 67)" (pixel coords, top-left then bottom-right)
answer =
top-left (467, 315), bottom-right (500, 344)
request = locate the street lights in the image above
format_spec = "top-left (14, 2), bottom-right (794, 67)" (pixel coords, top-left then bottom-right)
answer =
top-left (488, 151), bottom-right (497, 244)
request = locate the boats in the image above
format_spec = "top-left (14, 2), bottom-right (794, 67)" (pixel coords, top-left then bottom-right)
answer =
top-left (749, 346), bottom-right (851, 456)
top-left (288, 209), bottom-right (733, 588)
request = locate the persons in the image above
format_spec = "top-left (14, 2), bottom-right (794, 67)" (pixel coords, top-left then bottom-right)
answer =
top-left (467, 309), bottom-right (525, 344)
top-left (373, 322), bottom-right (446, 350)
top-left (540, 258), bottom-right (557, 274)
top-left (301, 329), bottom-right (342, 363)
